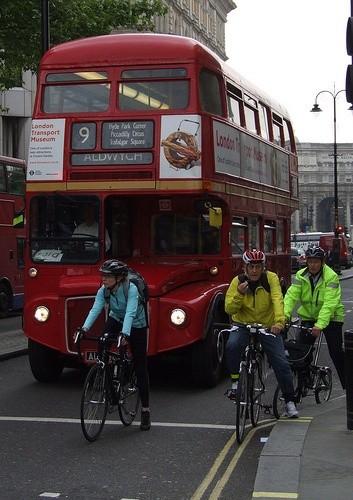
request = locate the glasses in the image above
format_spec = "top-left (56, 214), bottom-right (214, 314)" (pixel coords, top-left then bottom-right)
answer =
top-left (101, 274), bottom-right (114, 279)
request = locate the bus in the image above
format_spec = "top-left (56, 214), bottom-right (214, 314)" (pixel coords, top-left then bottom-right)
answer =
top-left (0, 156), bottom-right (27, 319)
top-left (13, 32), bottom-right (301, 388)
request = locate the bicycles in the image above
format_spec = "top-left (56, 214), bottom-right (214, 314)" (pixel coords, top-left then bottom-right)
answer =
top-left (213, 321), bottom-right (285, 444)
top-left (74, 326), bottom-right (141, 442)
top-left (273, 319), bottom-right (334, 421)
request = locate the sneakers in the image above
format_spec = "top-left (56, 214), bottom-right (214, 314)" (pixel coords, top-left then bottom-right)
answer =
top-left (285, 401), bottom-right (300, 418)
top-left (229, 381), bottom-right (238, 398)
top-left (140, 407), bottom-right (151, 431)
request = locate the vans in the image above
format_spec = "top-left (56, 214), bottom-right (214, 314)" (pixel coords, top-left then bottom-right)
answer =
top-left (291, 230), bottom-right (353, 274)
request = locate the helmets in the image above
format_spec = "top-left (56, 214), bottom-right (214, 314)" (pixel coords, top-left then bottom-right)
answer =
top-left (305, 247), bottom-right (325, 259)
top-left (242, 249), bottom-right (265, 265)
top-left (98, 258), bottom-right (128, 277)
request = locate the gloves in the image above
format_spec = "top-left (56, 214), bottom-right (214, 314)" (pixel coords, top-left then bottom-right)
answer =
top-left (72, 328), bottom-right (84, 340)
top-left (118, 333), bottom-right (130, 346)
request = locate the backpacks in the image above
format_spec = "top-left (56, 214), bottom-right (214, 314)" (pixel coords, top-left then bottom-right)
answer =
top-left (127, 267), bottom-right (149, 307)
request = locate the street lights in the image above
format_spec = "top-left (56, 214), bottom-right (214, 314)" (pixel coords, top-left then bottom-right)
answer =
top-left (309, 81), bottom-right (353, 275)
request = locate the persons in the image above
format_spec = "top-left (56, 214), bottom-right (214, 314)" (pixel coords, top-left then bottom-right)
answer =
top-left (73, 259), bottom-right (152, 431)
top-left (69, 206), bottom-right (111, 253)
top-left (223, 249), bottom-right (300, 418)
top-left (281, 243), bottom-right (347, 394)
